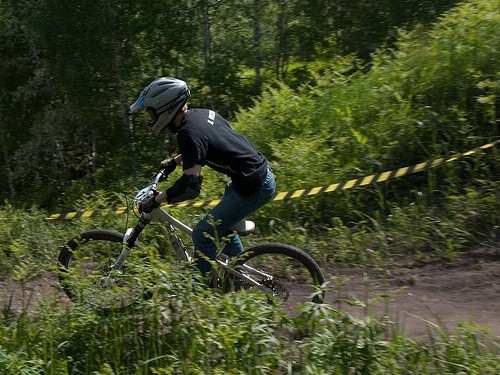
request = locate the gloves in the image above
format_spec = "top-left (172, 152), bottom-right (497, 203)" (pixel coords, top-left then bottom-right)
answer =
top-left (160, 158), bottom-right (178, 174)
top-left (138, 192), bottom-right (161, 214)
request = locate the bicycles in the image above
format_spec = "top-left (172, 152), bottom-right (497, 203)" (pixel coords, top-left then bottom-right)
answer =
top-left (57, 167), bottom-right (326, 317)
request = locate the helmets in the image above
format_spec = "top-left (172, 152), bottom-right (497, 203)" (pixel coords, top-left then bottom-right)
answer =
top-left (130, 77), bottom-right (191, 137)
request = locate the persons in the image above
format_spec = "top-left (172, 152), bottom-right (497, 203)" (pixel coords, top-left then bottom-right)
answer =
top-left (129, 76), bottom-right (278, 277)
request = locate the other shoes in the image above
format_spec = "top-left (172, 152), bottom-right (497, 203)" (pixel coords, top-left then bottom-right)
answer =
top-left (234, 274), bottom-right (250, 284)
